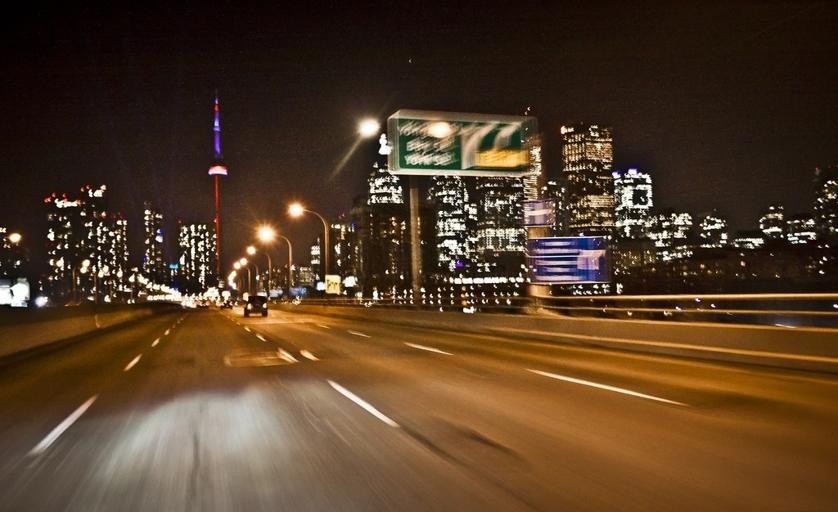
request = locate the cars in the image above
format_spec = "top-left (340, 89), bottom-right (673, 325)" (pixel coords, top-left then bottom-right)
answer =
top-left (196, 296), bottom-right (240, 310)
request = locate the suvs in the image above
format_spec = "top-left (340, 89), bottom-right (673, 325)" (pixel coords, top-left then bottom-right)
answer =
top-left (242, 296), bottom-right (268, 318)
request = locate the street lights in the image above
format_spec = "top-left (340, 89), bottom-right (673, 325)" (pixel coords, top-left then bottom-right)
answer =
top-left (70, 257), bottom-right (136, 305)
top-left (0, 230), bottom-right (23, 245)
top-left (353, 115), bottom-right (426, 310)
top-left (258, 222), bottom-right (294, 302)
top-left (233, 260), bottom-right (252, 295)
top-left (241, 256), bottom-right (261, 296)
top-left (245, 242), bottom-right (273, 300)
top-left (287, 202), bottom-right (332, 300)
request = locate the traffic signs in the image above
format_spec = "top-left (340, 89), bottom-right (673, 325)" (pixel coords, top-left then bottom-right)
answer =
top-left (524, 235), bottom-right (614, 285)
top-left (387, 108), bottom-right (530, 178)
